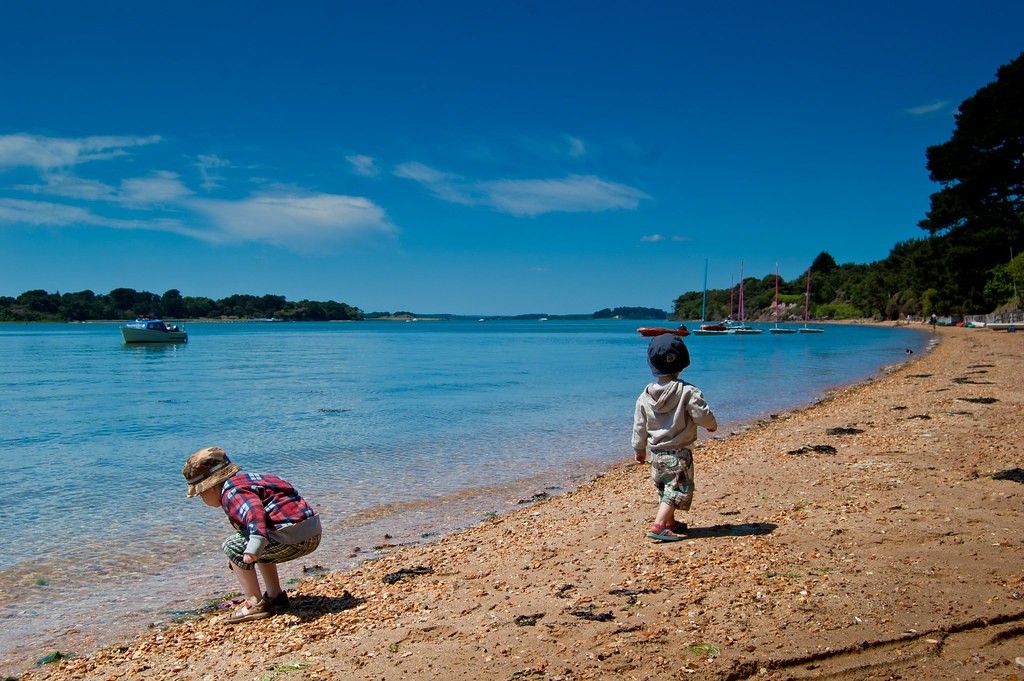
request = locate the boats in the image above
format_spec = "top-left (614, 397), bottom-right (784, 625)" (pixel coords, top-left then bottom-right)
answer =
top-left (637, 324), bottom-right (691, 336)
top-left (692, 257), bottom-right (729, 335)
top-left (119, 318), bottom-right (188, 343)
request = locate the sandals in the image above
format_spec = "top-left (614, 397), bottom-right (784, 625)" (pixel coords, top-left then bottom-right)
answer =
top-left (262, 590), bottom-right (290, 614)
top-left (646, 524), bottom-right (682, 540)
top-left (223, 600), bottom-right (270, 623)
top-left (666, 520), bottom-right (687, 532)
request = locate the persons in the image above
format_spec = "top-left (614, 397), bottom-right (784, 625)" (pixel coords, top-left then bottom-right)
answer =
top-left (631, 332), bottom-right (717, 540)
top-left (165, 322), bottom-right (170, 329)
top-left (183, 446), bottom-right (322, 622)
top-left (932, 312), bottom-right (938, 328)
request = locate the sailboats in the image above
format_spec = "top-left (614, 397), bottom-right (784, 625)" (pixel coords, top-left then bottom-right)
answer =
top-left (799, 267), bottom-right (825, 333)
top-left (720, 258), bottom-right (764, 334)
top-left (769, 261), bottom-right (797, 333)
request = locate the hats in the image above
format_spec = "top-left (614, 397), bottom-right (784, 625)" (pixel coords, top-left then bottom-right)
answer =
top-left (647, 334), bottom-right (690, 377)
top-left (182, 446), bottom-right (240, 498)
